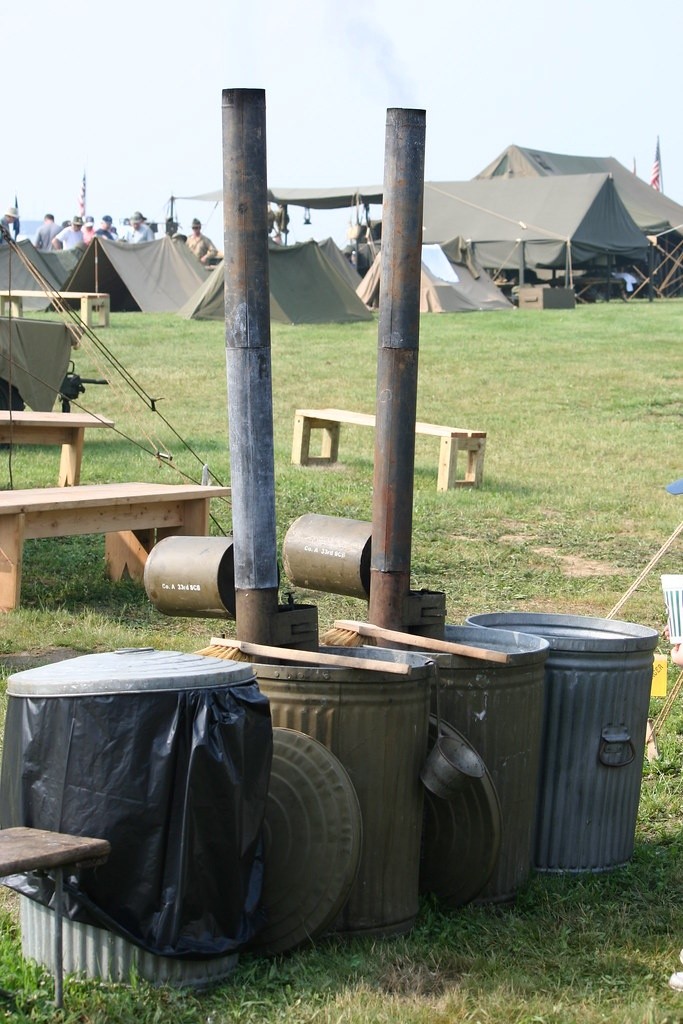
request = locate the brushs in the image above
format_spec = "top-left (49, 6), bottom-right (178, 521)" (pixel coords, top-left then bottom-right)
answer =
top-left (322, 616), bottom-right (511, 664)
top-left (193, 634), bottom-right (412, 677)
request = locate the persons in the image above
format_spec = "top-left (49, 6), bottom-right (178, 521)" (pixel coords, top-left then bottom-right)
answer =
top-left (0, 206), bottom-right (218, 265)
top-left (663, 479), bottom-right (683, 991)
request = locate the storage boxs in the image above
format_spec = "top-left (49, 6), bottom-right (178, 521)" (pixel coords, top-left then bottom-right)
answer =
top-left (518, 287), bottom-right (575, 309)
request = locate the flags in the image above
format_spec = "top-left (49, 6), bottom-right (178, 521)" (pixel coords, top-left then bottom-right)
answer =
top-left (651, 137), bottom-right (660, 192)
top-left (13, 193), bottom-right (20, 239)
top-left (78, 173), bottom-right (86, 217)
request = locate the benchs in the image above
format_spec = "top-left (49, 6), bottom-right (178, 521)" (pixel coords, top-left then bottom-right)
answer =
top-left (0, 480), bottom-right (232, 608)
top-left (0, 408), bottom-right (114, 490)
top-left (1, 825), bottom-right (109, 1010)
top-left (290, 405), bottom-right (486, 491)
top-left (2, 289), bottom-right (109, 328)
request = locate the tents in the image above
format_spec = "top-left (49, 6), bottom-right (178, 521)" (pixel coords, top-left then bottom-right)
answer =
top-left (169, 146), bottom-right (683, 325)
top-left (0, 236), bottom-right (213, 312)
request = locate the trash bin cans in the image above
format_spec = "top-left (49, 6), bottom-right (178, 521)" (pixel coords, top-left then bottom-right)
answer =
top-left (361, 623), bottom-right (550, 915)
top-left (1, 644), bottom-right (259, 990)
top-left (461, 609), bottom-right (660, 873)
top-left (250, 646), bottom-right (436, 958)
